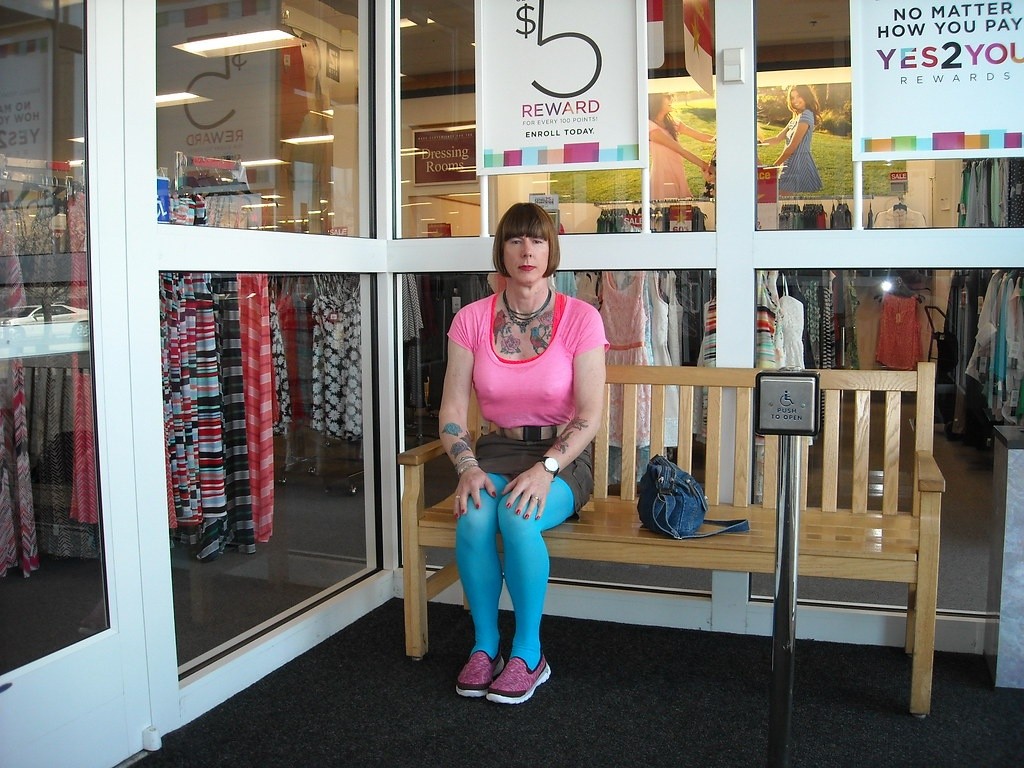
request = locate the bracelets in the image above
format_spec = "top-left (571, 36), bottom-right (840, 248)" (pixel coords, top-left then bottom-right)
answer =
top-left (453, 456), bottom-right (479, 479)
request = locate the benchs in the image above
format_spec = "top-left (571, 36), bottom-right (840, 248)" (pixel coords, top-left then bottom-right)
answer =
top-left (397, 355), bottom-right (944, 721)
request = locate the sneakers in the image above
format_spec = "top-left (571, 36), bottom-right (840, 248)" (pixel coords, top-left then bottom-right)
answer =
top-left (486, 646), bottom-right (551, 704)
top-left (456, 646), bottom-right (503, 697)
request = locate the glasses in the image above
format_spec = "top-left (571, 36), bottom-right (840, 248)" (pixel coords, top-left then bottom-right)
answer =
top-left (662, 93), bottom-right (676, 101)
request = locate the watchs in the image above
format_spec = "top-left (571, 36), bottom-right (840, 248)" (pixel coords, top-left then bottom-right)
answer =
top-left (537, 456), bottom-right (560, 479)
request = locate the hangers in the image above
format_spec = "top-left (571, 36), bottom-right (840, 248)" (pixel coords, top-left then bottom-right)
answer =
top-left (598, 199), bottom-right (707, 224)
top-left (0, 169), bottom-right (84, 212)
top-left (779, 195), bottom-right (852, 218)
top-left (869, 194), bottom-right (872, 213)
top-left (894, 194), bottom-right (906, 212)
top-left (158, 154), bottom-right (278, 207)
top-left (874, 277), bottom-right (926, 304)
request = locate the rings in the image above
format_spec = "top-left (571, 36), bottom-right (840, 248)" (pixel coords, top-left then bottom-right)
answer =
top-left (531, 496), bottom-right (539, 501)
top-left (454, 495), bottom-right (460, 498)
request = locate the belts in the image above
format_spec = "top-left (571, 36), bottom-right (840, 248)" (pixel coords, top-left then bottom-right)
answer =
top-left (486, 420), bottom-right (569, 441)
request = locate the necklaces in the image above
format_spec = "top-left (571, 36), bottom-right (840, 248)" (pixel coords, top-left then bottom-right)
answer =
top-left (502, 285), bottom-right (551, 334)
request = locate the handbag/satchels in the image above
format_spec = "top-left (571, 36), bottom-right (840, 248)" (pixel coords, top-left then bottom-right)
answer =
top-left (636, 454), bottom-right (749, 540)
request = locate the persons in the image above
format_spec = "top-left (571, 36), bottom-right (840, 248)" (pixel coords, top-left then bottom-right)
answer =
top-left (764, 85), bottom-right (824, 195)
top-left (648, 92), bottom-right (717, 200)
top-left (436, 201), bottom-right (610, 705)
